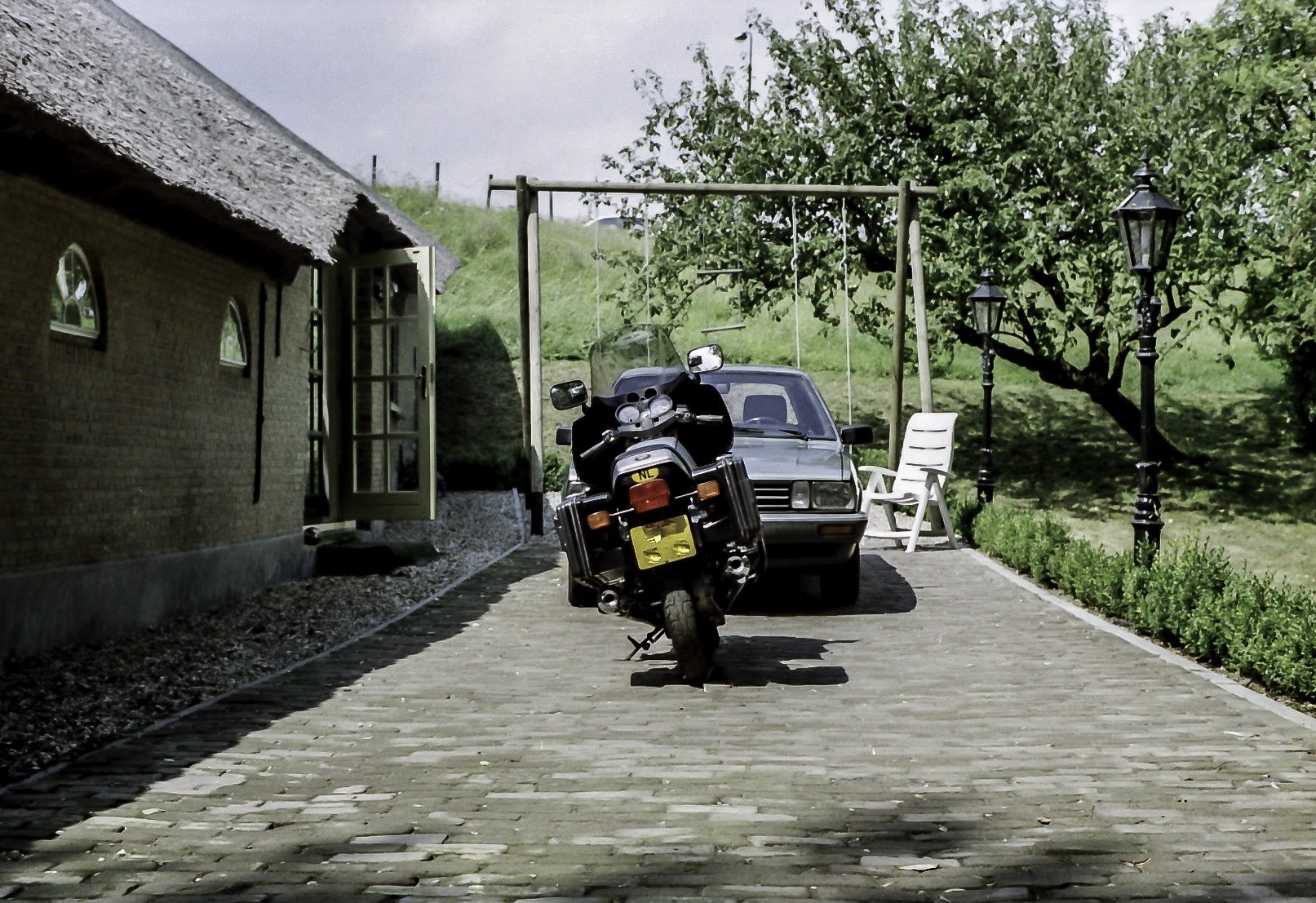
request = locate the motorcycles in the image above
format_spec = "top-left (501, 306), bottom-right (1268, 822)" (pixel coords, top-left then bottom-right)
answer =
top-left (550, 321), bottom-right (768, 682)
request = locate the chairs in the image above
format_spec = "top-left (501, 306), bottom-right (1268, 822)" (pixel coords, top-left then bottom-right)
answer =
top-left (743, 395), bottom-right (787, 424)
top-left (858, 413), bottom-right (959, 553)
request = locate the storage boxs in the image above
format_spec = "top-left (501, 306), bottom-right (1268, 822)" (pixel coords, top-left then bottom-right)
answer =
top-left (553, 494), bottom-right (617, 579)
top-left (691, 456), bottom-right (762, 542)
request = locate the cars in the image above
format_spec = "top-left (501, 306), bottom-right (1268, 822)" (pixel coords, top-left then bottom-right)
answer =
top-left (610, 365), bottom-right (868, 605)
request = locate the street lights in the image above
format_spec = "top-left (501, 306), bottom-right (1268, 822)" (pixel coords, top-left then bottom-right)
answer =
top-left (968, 268), bottom-right (1008, 507)
top-left (1109, 148), bottom-right (1187, 567)
